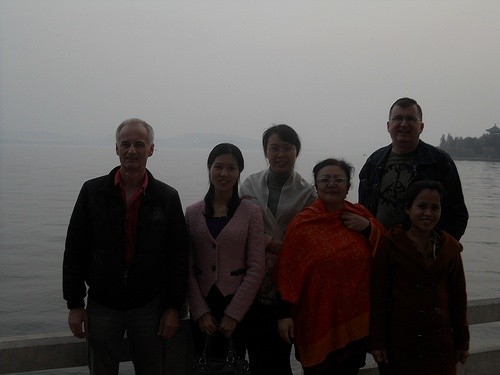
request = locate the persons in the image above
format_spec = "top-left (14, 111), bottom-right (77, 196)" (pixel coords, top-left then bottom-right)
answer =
top-left (241, 125), bottom-right (318, 375)
top-left (359, 98), bottom-right (468, 241)
top-left (276, 159), bottom-right (386, 375)
top-left (184, 144), bottom-right (266, 360)
top-left (62, 118), bottom-right (188, 375)
top-left (367, 179), bottom-right (470, 375)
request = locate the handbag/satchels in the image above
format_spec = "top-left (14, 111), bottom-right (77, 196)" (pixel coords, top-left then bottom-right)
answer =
top-left (190, 329), bottom-right (249, 375)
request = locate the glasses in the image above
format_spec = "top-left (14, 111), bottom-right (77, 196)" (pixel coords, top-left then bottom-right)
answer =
top-left (318, 178), bottom-right (348, 185)
